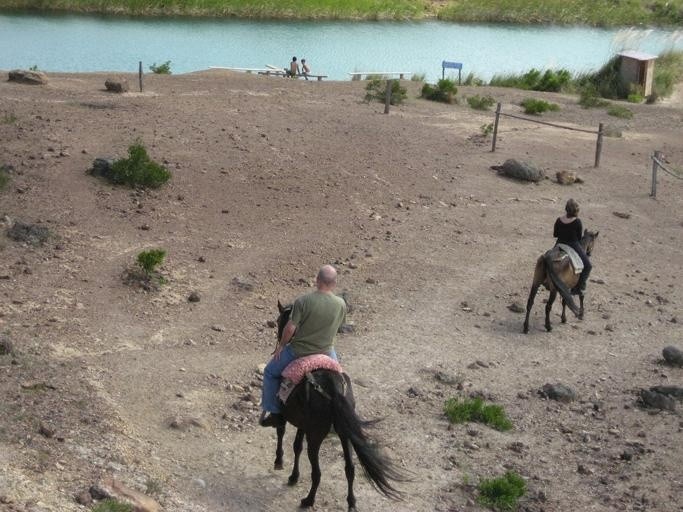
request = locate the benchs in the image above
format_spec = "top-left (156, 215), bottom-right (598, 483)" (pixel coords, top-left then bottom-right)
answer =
top-left (208, 65), bottom-right (330, 83)
top-left (348, 71), bottom-right (413, 82)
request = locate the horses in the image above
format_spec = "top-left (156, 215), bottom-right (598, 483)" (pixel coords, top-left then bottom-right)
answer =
top-left (522, 226), bottom-right (601, 334)
top-left (268, 296), bottom-right (412, 512)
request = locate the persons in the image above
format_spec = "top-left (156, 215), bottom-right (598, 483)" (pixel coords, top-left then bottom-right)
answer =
top-left (258, 265), bottom-right (348, 426)
top-left (283, 56), bottom-right (300, 78)
top-left (552, 197), bottom-right (592, 296)
top-left (300, 59), bottom-right (310, 81)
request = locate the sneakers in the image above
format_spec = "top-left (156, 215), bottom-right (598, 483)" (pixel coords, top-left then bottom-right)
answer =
top-left (571, 286), bottom-right (583, 295)
top-left (261, 412), bottom-right (287, 427)
